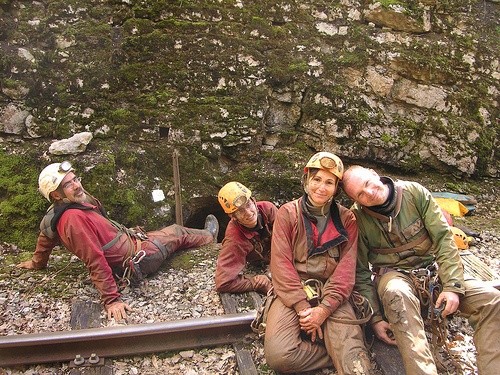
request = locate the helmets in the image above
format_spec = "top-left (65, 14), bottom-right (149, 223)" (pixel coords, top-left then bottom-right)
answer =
top-left (304, 152), bottom-right (344, 181)
top-left (218, 182), bottom-right (251, 213)
top-left (39, 163), bottom-right (75, 203)
top-left (450, 226), bottom-right (468, 249)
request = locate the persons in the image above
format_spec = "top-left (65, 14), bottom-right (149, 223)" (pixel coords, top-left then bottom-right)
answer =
top-left (264, 152), bottom-right (374, 375)
top-left (16, 163), bottom-right (219, 322)
top-left (342, 166), bottom-right (499, 375)
top-left (215, 182), bottom-right (278, 295)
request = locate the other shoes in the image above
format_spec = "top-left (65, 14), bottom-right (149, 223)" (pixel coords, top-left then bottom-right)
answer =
top-left (204, 214), bottom-right (219, 244)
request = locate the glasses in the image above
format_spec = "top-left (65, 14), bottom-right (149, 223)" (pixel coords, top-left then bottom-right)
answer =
top-left (58, 177), bottom-right (81, 190)
